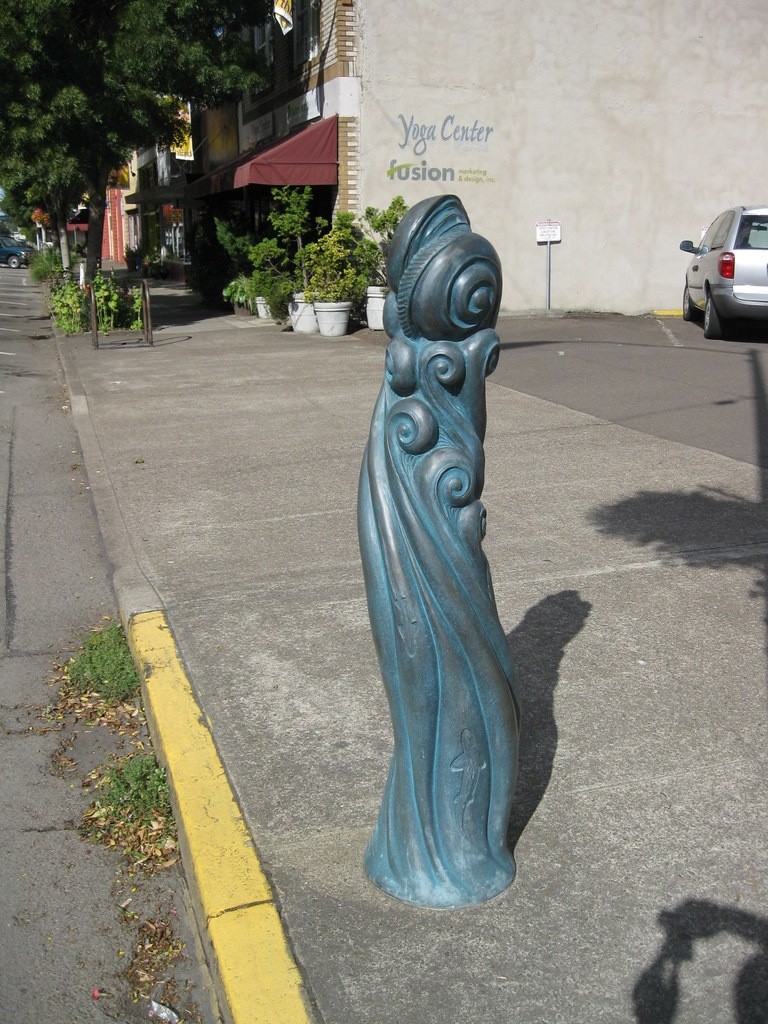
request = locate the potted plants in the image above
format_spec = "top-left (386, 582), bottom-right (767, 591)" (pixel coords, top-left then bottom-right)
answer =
top-left (212, 183), bottom-right (409, 338)
top-left (124, 243), bottom-right (174, 279)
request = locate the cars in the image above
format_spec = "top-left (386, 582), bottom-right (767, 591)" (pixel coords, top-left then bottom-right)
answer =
top-left (0, 235), bottom-right (34, 269)
top-left (680, 205), bottom-right (768, 339)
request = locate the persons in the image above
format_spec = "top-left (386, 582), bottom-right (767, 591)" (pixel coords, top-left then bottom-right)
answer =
top-left (75, 243), bottom-right (84, 256)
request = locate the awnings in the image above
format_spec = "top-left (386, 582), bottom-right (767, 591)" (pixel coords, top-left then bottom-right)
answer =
top-left (126, 186), bottom-right (186, 203)
top-left (184, 115), bottom-right (339, 200)
